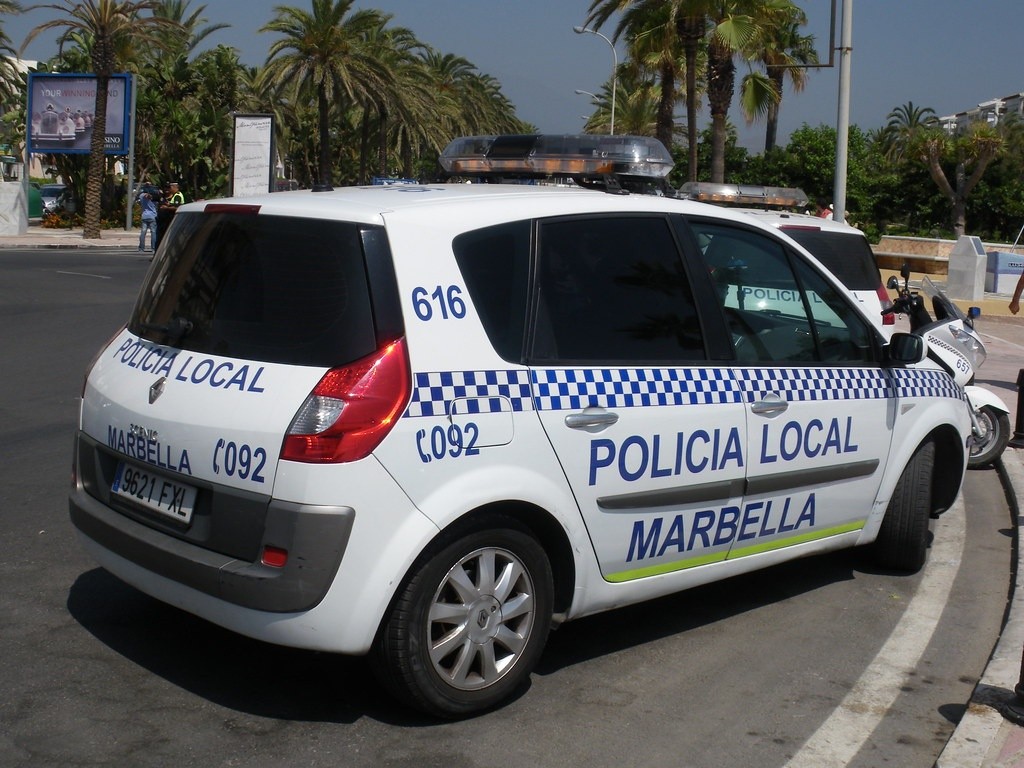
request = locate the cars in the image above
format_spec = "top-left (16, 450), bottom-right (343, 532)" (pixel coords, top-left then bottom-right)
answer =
top-left (40, 184), bottom-right (76, 218)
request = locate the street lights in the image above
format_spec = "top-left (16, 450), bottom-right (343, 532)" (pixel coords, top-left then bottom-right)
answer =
top-left (574, 90), bottom-right (602, 119)
top-left (573, 26), bottom-right (617, 135)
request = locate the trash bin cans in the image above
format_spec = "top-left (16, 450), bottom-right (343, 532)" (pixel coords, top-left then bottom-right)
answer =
top-left (945, 235), bottom-right (988, 302)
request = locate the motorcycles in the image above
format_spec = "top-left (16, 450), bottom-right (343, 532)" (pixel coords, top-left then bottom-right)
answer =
top-left (881, 262), bottom-right (1011, 469)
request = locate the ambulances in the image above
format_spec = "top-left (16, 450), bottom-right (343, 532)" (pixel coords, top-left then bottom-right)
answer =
top-left (70, 135), bottom-right (971, 719)
top-left (673, 182), bottom-right (895, 350)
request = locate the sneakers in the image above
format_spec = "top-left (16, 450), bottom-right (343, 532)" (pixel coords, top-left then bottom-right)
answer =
top-left (152, 250), bottom-right (155, 255)
top-left (137, 248), bottom-right (145, 253)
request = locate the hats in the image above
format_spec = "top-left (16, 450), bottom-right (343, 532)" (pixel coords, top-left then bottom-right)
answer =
top-left (165, 181), bottom-right (180, 185)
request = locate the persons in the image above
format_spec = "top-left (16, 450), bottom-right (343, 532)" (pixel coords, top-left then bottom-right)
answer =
top-left (135, 182), bottom-right (184, 254)
top-left (1009, 271), bottom-right (1024, 315)
top-left (816, 198), bottom-right (832, 218)
